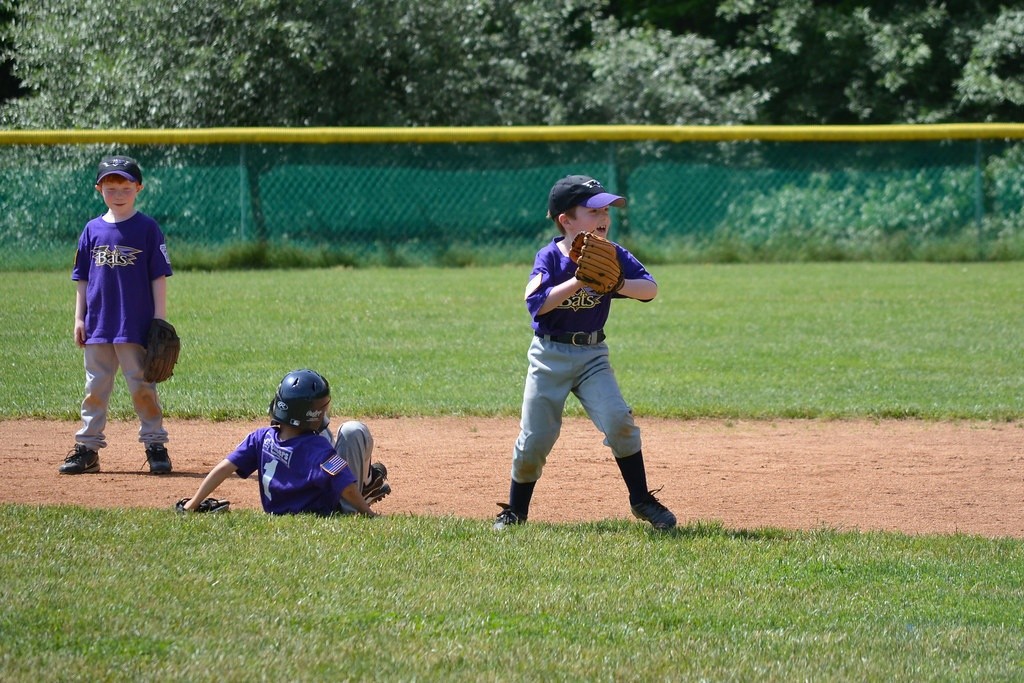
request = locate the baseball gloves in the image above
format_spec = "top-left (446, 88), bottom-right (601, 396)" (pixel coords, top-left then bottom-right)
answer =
top-left (568, 230), bottom-right (624, 296)
top-left (175, 497), bottom-right (231, 515)
top-left (142, 318), bottom-right (181, 384)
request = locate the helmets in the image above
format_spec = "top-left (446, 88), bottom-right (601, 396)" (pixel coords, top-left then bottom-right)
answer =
top-left (270, 369), bottom-right (331, 431)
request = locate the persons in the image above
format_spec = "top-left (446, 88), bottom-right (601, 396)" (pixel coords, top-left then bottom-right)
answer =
top-left (60, 156), bottom-right (181, 474)
top-left (176, 369), bottom-right (391, 516)
top-left (492, 174), bottom-right (677, 531)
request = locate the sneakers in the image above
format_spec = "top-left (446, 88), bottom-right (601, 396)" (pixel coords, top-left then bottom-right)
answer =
top-left (492, 503), bottom-right (529, 535)
top-left (58, 444), bottom-right (101, 475)
top-left (360, 462), bottom-right (387, 498)
top-left (628, 485), bottom-right (676, 530)
top-left (140, 443), bottom-right (172, 474)
top-left (362, 483), bottom-right (391, 507)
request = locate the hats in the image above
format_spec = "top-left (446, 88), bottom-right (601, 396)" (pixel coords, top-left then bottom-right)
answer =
top-left (547, 175), bottom-right (626, 221)
top-left (96, 156), bottom-right (142, 186)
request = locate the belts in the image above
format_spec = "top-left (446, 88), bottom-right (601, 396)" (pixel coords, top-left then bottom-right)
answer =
top-left (535, 329), bottom-right (606, 346)
